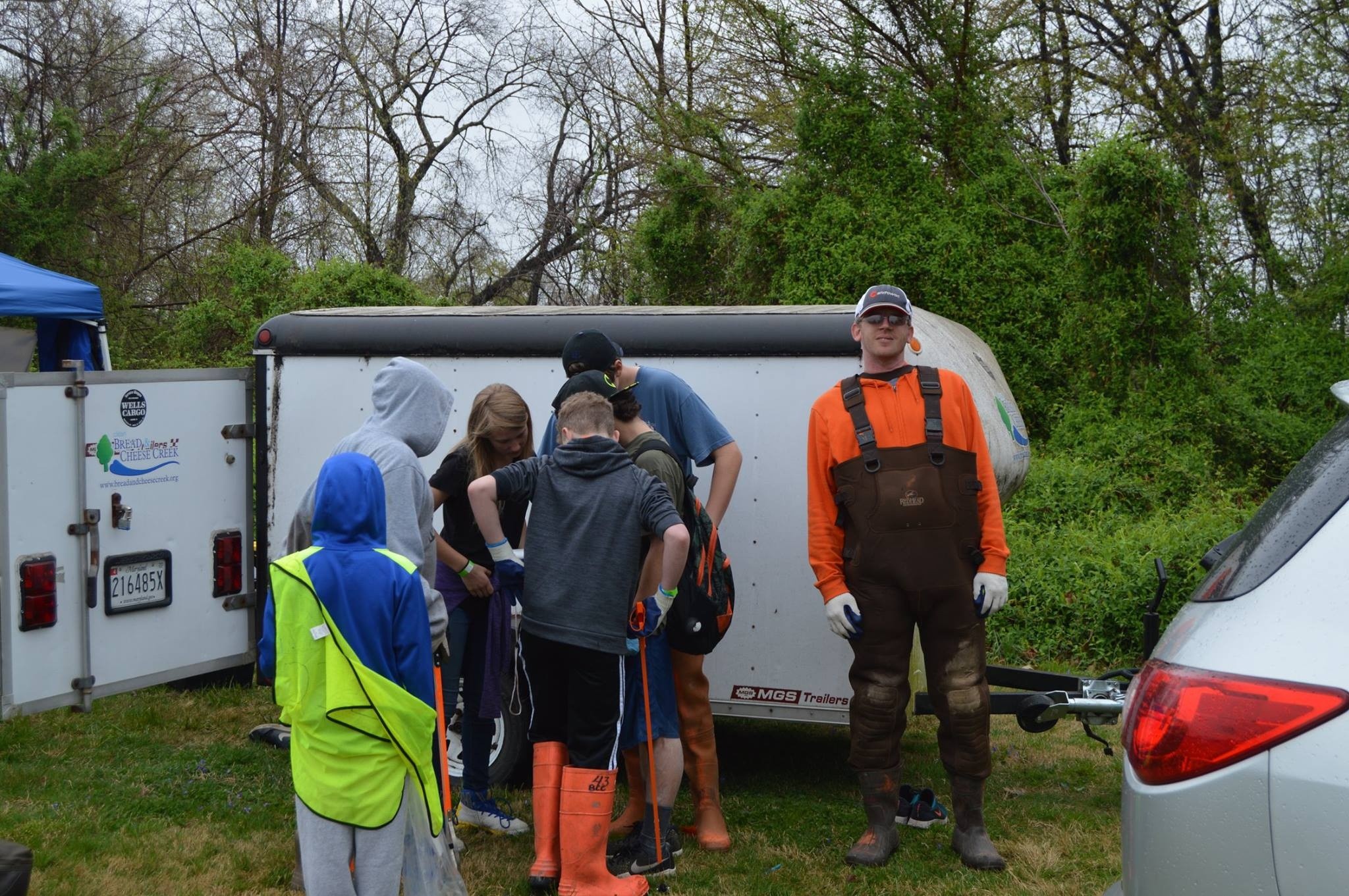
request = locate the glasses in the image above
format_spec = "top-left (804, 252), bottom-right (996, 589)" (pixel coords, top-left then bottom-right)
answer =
top-left (856, 311), bottom-right (910, 326)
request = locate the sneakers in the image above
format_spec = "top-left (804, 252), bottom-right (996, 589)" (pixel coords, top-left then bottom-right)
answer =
top-left (248, 723), bottom-right (291, 750)
top-left (895, 784), bottom-right (948, 830)
top-left (606, 821), bottom-right (683, 879)
top-left (454, 787), bottom-right (531, 836)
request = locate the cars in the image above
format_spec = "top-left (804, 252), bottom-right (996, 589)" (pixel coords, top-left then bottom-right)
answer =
top-left (1105, 378), bottom-right (1349, 895)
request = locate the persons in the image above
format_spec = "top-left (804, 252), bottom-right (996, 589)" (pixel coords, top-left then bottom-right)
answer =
top-left (287, 357), bottom-right (464, 851)
top-left (255, 453), bottom-right (449, 896)
top-left (539, 330), bottom-right (744, 852)
top-left (466, 392), bottom-right (689, 896)
top-left (805, 282), bottom-right (1011, 874)
top-left (424, 383), bottom-right (531, 839)
top-left (552, 370), bottom-right (687, 884)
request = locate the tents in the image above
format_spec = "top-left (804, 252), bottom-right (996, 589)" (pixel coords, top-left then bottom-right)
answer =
top-left (0, 250), bottom-right (113, 373)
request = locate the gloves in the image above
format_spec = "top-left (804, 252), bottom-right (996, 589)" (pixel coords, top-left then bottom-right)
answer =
top-left (485, 537), bottom-right (525, 608)
top-left (627, 582), bottom-right (678, 639)
top-left (824, 592), bottom-right (865, 640)
top-left (973, 572), bottom-right (1009, 619)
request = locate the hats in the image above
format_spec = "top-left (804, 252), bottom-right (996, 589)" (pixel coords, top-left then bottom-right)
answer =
top-left (551, 369), bottom-right (640, 420)
top-left (855, 284), bottom-right (912, 321)
top-left (562, 328), bottom-right (624, 377)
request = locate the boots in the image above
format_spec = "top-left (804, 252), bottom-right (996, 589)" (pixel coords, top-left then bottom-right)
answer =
top-left (948, 771), bottom-right (1006, 872)
top-left (556, 763), bottom-right (650, 896)
top-left (844, 762), bottom-right (905, 867)
top-left (606, 741), bottom-right (650, 836)
top-left (528, 741), bottom-right (569, 892)
top-left (669, 646), bottom-right (732, 853)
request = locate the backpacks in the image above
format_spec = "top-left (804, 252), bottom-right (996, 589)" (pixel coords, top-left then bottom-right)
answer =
top-left (631, 439), bottom-right (736, 655)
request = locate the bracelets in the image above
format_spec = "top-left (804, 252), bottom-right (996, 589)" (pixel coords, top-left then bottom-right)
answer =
top-left (458, 560), bottom-right (476, 578)
